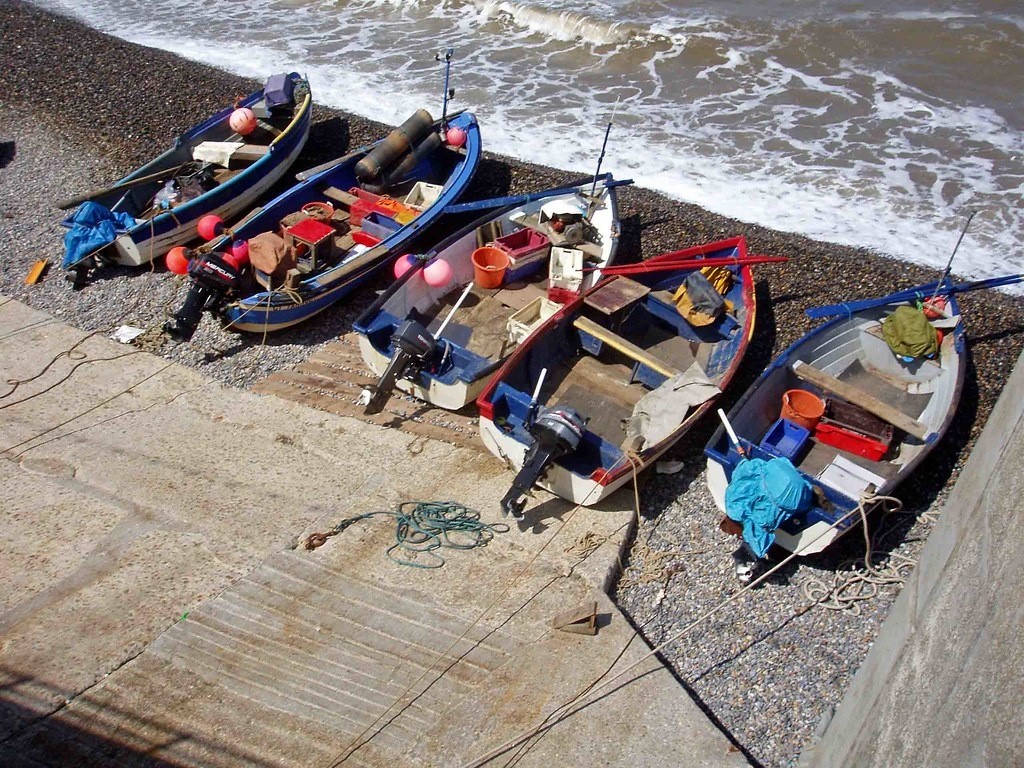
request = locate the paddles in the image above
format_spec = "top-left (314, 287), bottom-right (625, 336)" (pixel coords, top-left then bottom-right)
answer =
top-left (443, 179), bottom-right (635, 215)
top-left (597, 253), bottom-right (790, 277)
top-left (803, 272), bottom-right (1024, 321)
top-left (57, 161), bottom-right (195, 210)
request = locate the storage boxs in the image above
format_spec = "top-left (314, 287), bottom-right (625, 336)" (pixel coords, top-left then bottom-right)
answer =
top-left (347, 186), bottom-right (380, 204)
top-left (549, 247), bottom-right (585, 289)
top-left (502, 259), bottom-right (548, 285)
top-left (504, 295), bottom-right (563, 344)
top-left (547, 286), bottom-right (581, 303)
top-left (254, 100), bottom-right (272, 120)
top-left (816, 396), bottom-right (894, 461)
top-left (492, 226), bottom-right (551, 257)
top-left (264, 72), bottom-right (295, 106)
top-left (360, 213), bottom-right (405, 237)
top-left (762, 417), bottom-right (811, 462)
top-left (486, 243), bottom-right (550, 269)
top-left (403, 181), bottom-right (444, 211)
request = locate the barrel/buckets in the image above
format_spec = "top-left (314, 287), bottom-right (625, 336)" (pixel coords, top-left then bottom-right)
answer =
top-left (303, 202), bottom-right (334, 229)
top-left (472, 244), bottom-right (509, 287)
top-left (779, 390), bottom-right (826, 432)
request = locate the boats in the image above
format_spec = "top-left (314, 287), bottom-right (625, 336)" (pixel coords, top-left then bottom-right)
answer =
top-left (57, 71), bottom-right (312, 293)
top-left (472, 235), bottom-right (757, 524)
top-left (162, 46), bottom-right (482, 346)
top-left (704, 208), bottom-right (979, 563)
top-left (350, 93), bottom-right (638, 414)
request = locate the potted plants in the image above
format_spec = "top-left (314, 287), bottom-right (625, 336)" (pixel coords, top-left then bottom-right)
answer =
top-left (303, 201), bottom-right (335, 226)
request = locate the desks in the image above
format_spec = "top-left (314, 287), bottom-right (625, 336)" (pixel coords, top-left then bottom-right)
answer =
top-left (587, 274), bottom-right (652, 332)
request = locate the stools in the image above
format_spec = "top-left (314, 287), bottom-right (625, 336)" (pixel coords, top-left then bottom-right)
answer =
top-left (285, 219), bottom-right (339, 272)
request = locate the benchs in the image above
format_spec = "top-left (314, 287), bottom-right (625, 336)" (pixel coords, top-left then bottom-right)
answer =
top-left (324, 186), bottom-right (414, 227)
top-left (792, 361), bottom-right (938, 444)
top-left (572, 316), bottom-right (681, 389)
